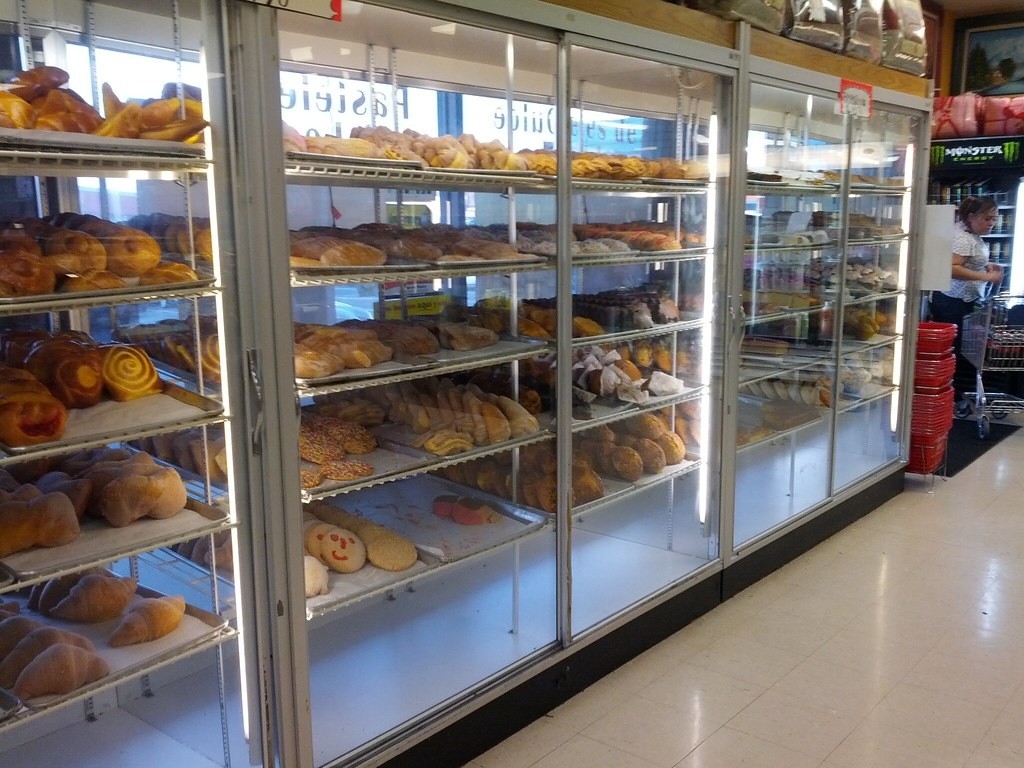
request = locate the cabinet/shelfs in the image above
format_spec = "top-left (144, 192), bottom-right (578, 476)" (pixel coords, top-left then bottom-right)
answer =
top-left (0, 123), bottom-right (912, 735)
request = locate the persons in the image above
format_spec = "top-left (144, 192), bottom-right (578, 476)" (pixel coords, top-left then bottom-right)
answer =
top-left (927, 196), bottom-right (1004, 416)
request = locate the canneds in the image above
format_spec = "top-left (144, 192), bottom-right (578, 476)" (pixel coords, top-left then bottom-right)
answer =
top-left (940, 182), bottom-right (1015, 259)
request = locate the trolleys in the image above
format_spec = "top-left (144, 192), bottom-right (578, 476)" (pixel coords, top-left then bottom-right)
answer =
top-left (954, 273), bottom-right (1024, 442)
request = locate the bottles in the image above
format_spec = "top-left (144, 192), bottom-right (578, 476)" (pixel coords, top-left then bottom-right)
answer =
top-left (974, 325), bottom-right (1024, 368)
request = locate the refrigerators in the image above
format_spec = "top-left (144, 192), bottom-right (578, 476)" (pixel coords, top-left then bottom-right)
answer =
top-left (917, 134), bottom-right (1024, 430)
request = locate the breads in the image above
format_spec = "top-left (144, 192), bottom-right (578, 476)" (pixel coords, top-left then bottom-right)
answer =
top-left (279, 121), bottom-right (713, 600)
top-left (735, 165), bottom-right (904, 450)
top-left (0, 68), bottom-right (234, 703)
